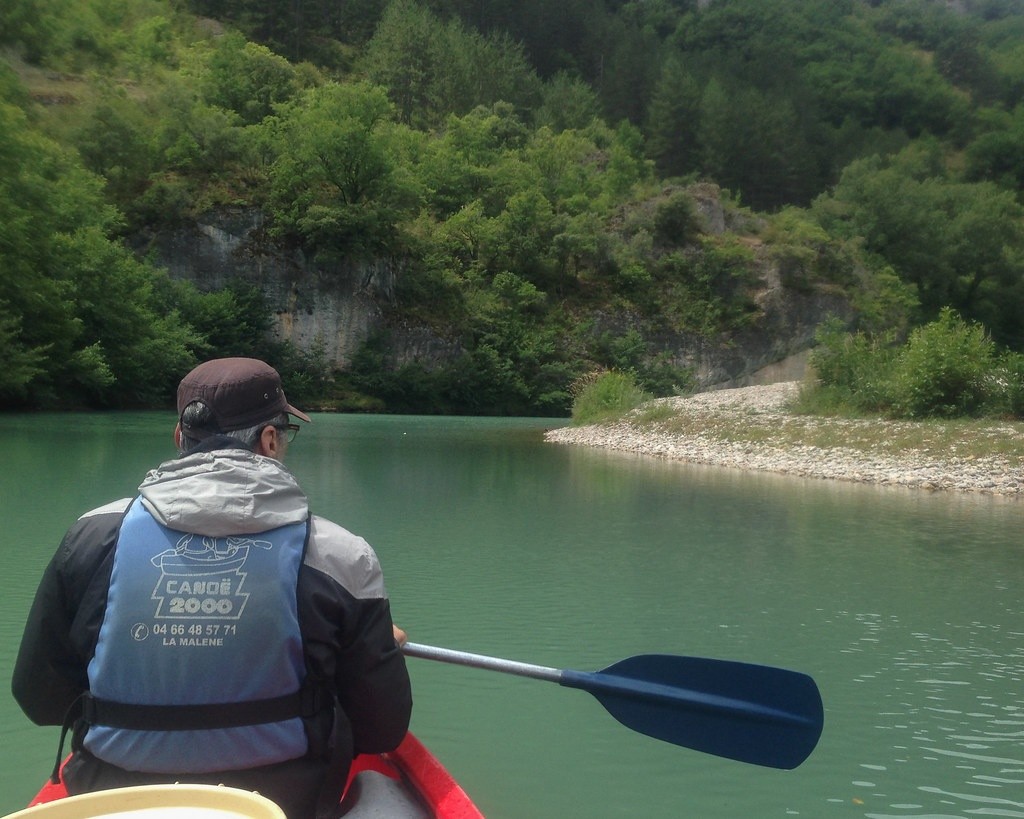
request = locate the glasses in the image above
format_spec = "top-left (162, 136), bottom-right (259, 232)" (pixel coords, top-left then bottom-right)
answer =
top-left (276, 422), bottom-right (300, 440)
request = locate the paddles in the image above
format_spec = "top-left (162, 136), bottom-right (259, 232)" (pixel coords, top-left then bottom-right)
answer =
top-left (401, 640), bottom-right (824, 772)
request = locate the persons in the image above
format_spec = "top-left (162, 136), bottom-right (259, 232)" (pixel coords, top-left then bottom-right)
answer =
top-left (11, 357), bottom-right (412, 819)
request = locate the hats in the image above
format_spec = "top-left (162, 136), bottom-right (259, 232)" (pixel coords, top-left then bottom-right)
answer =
top-left (176, 358), bottom-right (311, 442)
